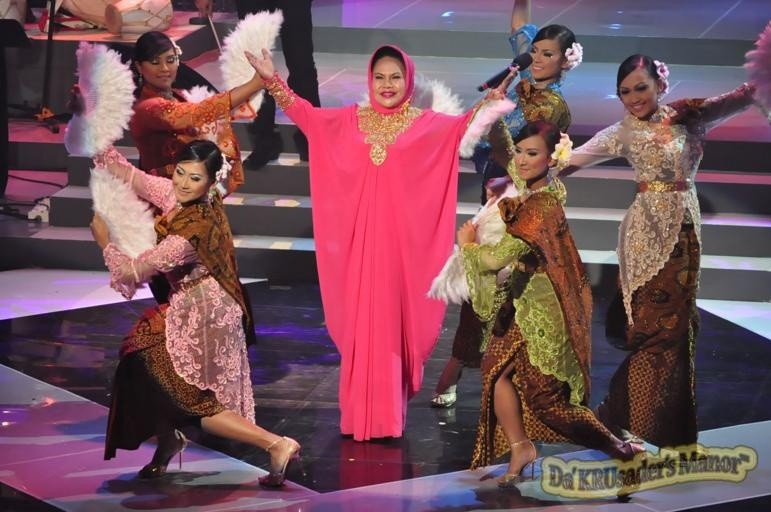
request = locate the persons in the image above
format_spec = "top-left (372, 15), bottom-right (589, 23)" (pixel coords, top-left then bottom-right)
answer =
top-left (430, 1), bottom-right (582, 408)
top-left (454, 88), bottom-right (658, 500)
top-left (245, 46), bottom-right (520, 443)
top-left (551, 55), bottom-right (767, 451)
top-left (192, 0), bottom-right (321, 170)
top-left (67, 78), bottom-right (302, 488)
top-left (129, 32), bottom-right (272, 337)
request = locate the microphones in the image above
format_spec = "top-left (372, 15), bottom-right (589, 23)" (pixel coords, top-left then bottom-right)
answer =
top-left (478, 53), bottom-right (533, 91)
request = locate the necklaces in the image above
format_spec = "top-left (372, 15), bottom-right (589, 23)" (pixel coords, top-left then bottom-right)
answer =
top-left (354, 102), bottom-right (422, 166)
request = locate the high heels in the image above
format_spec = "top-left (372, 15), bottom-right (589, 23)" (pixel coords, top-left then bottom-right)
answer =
top-left (432, 370), bottom-right (462, 407)
top-left (259, 436), bottom-right (306, 487)
top-left (498, 439), bottom-right (541, 488)
top-left (615, 440), bottom-right (648, 498)
top-left (139, 431), bottom-right (187, 479)
top-left (592, 404), bottom-right (645, 443)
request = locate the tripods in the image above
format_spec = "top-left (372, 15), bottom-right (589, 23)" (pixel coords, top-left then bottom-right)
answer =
top-left (7, 1), bottom-right (73, 132)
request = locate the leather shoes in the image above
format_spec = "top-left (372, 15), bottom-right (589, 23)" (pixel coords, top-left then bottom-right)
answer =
top-left (242, 149), bottom-right (280, 170)
top-left (299, 148), bottom-right (309, 162)
